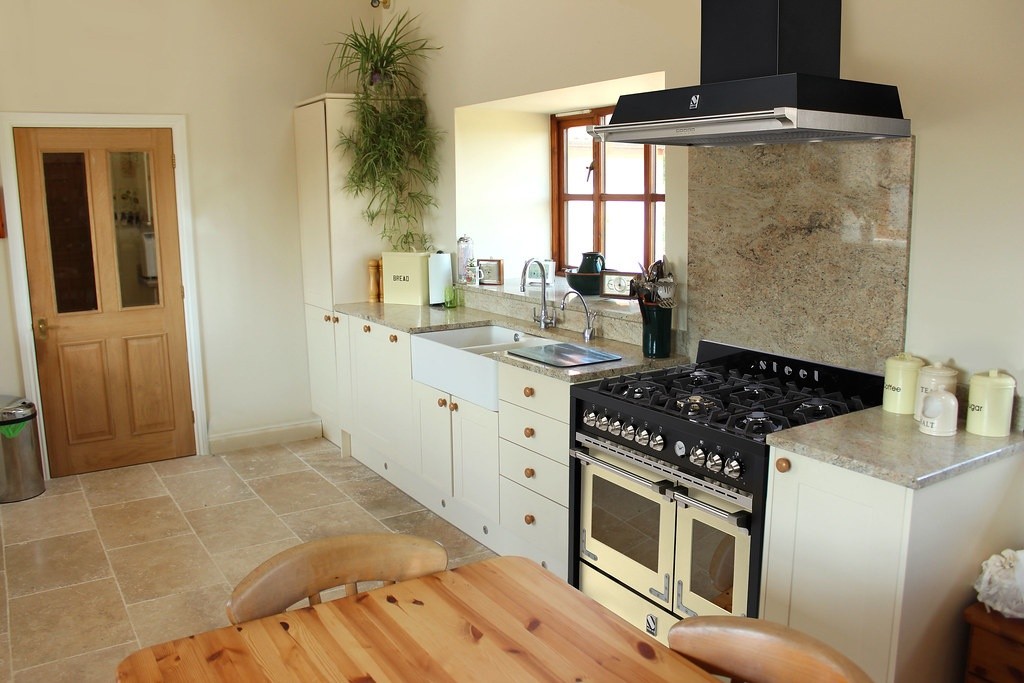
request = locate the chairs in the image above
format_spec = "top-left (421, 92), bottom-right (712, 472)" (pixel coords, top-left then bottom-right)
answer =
top-left (668, 615), bottom-right (875, 683)
top-left (227, 532), bottom-right (448, 630)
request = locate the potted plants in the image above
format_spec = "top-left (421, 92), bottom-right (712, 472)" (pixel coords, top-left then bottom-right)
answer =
top-left (326, 9), bottom-right (446, 252)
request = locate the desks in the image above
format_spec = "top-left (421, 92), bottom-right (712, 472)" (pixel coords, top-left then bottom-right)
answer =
top-left (114, 556), bottom-right (722, 682)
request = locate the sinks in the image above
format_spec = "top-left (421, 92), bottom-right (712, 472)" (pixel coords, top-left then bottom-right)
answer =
top-left (459, 339), bottom-right (563, 412)
top-left (410, 325), bottom-right (532, 397)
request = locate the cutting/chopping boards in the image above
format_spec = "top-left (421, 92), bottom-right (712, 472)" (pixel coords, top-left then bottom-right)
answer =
top-left (507, 342), bottom-right (622, 368)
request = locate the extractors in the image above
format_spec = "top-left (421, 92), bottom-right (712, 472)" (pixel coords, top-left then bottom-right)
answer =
top-left (587, 0), bottom-right (912, 146)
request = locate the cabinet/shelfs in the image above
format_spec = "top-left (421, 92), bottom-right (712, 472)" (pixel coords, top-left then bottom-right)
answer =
top-left (499, 361), bottom-right (570, 582)
top-left (963, 602), bottom-right (1024, 683)
top-left (764, 446), bottom-right (910, 683)
top-left (304, 305), bottom-right (499, 522)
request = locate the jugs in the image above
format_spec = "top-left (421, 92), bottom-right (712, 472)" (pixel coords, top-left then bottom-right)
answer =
top-left (577, 251), bottom-right (605, 273)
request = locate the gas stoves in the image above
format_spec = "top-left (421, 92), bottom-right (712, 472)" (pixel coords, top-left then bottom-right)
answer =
top-left (570, 339), bottom-right (884, 491)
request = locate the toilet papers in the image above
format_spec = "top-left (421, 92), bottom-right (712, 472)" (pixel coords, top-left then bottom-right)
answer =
top-left (428, 252), bottom-right (452, 304)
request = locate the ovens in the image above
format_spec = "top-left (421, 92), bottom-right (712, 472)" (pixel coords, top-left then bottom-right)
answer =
top-left (569, 431), bottom-right (764, 650)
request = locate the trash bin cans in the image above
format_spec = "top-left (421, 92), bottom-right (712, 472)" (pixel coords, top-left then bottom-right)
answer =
top-left (0, 395), bottom-right (46, 505)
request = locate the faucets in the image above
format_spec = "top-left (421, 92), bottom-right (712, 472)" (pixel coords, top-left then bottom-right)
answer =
top-left (559, 291), bottom-right (597, 340)
top-left (519, 257), bottom-right (556, 329)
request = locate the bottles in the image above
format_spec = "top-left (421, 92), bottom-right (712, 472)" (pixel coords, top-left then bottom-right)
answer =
top-left (444, 280), bottom-right (457, 307)
top-left (882, 352), bottom-right (1016, 438)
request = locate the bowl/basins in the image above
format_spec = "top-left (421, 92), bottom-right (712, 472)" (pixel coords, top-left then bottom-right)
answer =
top-left (565, 268), bottom-right (618, 295)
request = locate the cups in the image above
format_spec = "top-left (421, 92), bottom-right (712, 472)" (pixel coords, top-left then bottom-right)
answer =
top-left (637, 298), bottom-right (672, 359)
top-left (466, 266), bottom-right (484, 287)
